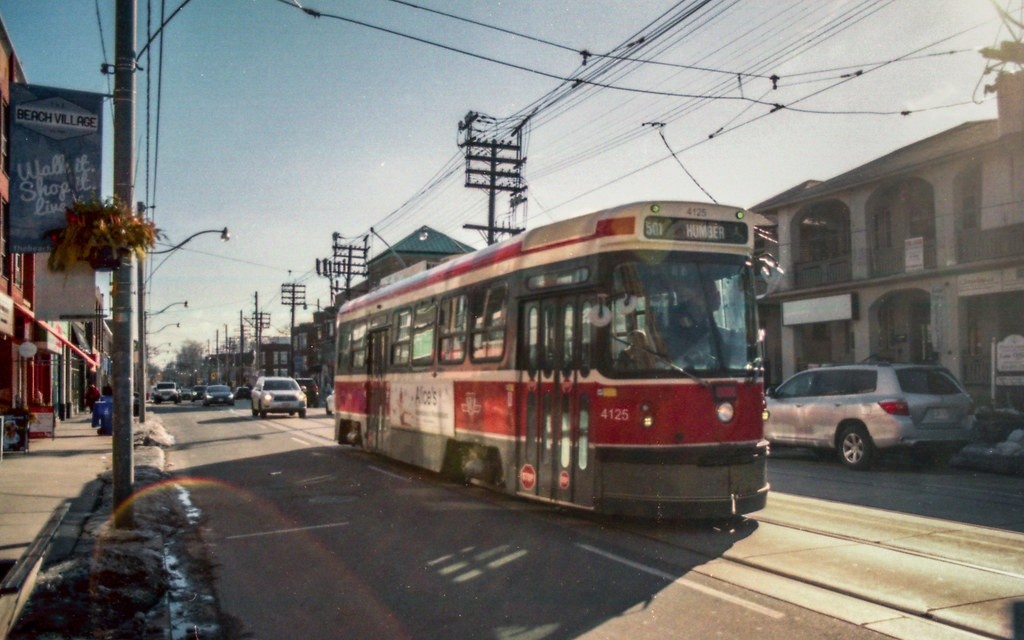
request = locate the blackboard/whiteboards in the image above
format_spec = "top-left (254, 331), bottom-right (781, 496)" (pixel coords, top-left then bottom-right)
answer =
top-left (996, 334), bottom-right (1024, 372)
top-left (0, 410), bottom-right (27, 453)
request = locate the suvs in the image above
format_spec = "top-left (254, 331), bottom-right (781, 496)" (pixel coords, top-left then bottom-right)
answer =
top-left (293, 377), bottom-right (319, 408)
top-left (251, 376), bottom-right (308, 419)
top-left (153, 381), bottom-right (183, 405)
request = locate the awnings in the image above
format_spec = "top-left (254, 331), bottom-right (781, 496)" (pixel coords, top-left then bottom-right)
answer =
top-left (13, 302), bottom-right (100, 368)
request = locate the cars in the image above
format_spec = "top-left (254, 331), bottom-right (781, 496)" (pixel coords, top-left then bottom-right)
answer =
top-left (181, 388), bottom-right (192, 401)
top-left (201, 384), bottom-right (235, 406)
top-left (190, 385), bottom-right (207, 403)
top-left (234, 387), bottom-right (251, 401)
top-left (325, 389), bottom-right (334, 415)
top-left (763, 359), bottom-right (987, 473)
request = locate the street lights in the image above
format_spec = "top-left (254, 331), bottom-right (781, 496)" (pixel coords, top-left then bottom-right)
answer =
top-left (138, 200), bottom-right (230, 424)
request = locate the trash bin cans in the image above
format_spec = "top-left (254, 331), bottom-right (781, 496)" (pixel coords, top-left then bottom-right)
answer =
top-left (91, 397), bottom-right (112, 436)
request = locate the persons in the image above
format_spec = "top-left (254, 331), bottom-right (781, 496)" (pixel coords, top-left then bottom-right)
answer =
top-left (86, 384), bottom-right (101, 412)
top-left (102, 383), bottom-right (112, 396)
top-left (618, 329), bottom-right (649, 367)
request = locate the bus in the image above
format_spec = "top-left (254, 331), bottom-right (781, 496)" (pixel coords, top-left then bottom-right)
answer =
top-left (334, 199), bottom-right (782, 523)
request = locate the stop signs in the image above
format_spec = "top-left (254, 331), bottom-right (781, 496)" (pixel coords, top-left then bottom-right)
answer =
top-left (520, 464), bottom-right (537, 489)
top-left (559, 470), bottom-right (570, 491)
